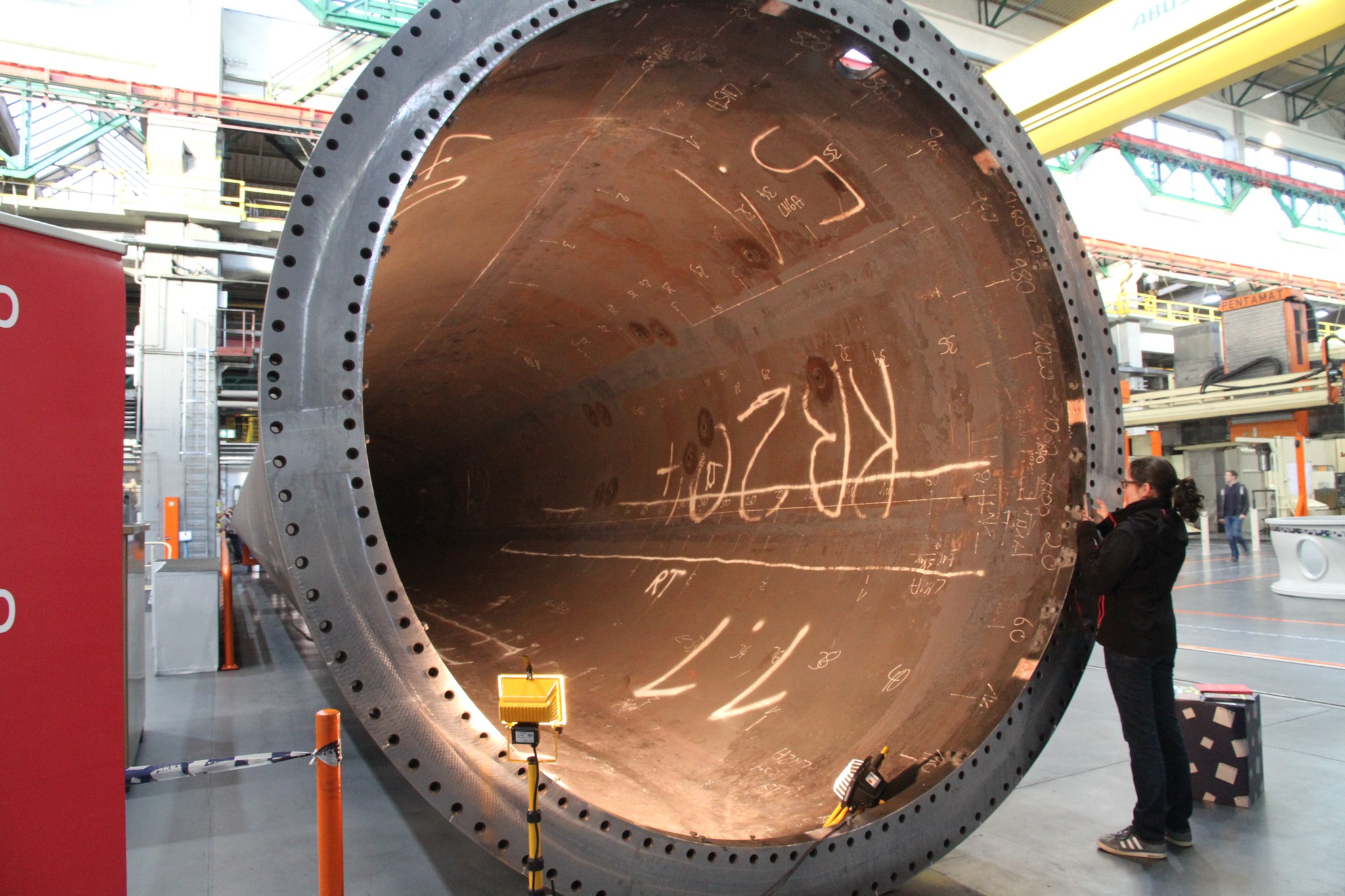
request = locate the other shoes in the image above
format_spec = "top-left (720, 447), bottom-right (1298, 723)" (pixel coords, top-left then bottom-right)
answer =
top-left (1226, 555), bottom-right (1239, 563)
top-left (1242, 543), bottom-right (1249, 552)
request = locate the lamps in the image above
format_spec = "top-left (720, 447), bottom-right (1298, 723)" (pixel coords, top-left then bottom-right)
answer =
top-left (493, 675), bottom-right (566, 724)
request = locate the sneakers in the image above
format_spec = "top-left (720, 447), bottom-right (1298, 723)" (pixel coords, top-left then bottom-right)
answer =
top-left (1164, 829), bottom-right (1194, 847)
top-left (1098, 826), bottom-right (1168, 859)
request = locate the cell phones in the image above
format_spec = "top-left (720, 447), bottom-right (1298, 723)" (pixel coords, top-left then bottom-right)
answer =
top-left (1083, 493), bottom-right (1093, 519)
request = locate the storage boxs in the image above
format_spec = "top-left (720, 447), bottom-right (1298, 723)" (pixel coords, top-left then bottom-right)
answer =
top-left (1173, 688), bottom-right (1265, 809)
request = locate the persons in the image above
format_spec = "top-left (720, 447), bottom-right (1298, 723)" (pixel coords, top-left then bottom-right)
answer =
top-left (1218, 469), bottom-right (1249, 563)
top-left (1074, 455), bottom-right (1204, 859)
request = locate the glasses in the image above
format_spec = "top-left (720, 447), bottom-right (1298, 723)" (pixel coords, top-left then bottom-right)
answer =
top-left (1121, 479), bottom-right (1143, 489)
